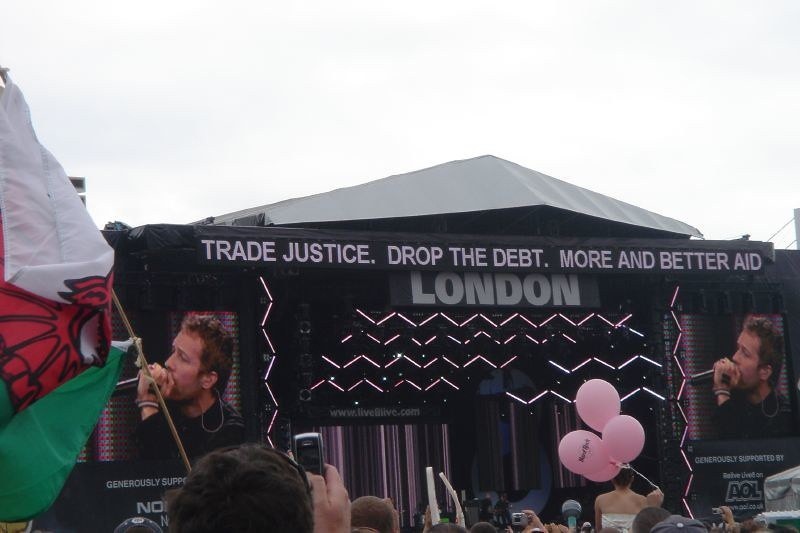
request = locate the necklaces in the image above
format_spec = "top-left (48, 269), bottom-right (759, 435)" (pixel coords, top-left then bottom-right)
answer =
top-left (356, 525), bottom-right (378, 532)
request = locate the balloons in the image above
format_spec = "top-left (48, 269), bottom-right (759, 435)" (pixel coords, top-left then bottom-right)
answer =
top-left (601, 413), bottom-right (646, 465)
top-left (574, 377), bottom-right (624, 433)
top-left (581, 460), bottom-right (624, 482)
top-left (556, 429), bottom-right (614, 474)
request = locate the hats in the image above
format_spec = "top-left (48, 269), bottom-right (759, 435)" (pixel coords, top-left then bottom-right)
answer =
top-left (649, 513), bottom-right (710, 533)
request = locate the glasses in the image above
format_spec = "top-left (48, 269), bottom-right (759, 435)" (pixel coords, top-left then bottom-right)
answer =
top-left (205, 438), bottom-right (315, 514)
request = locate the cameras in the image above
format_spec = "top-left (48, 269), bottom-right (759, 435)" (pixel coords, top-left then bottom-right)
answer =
top-left (510, 512), bottom-right (533, 525)
top-left (713, 507), bottom-right (725, 515)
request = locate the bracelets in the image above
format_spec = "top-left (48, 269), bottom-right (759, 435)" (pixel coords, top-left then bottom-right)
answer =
top-left (713, 387), bottom-right (731, 396)
top-left (137, 399), bottom-right (158, 410)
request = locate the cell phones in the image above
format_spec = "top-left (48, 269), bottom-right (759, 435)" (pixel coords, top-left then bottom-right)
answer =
top-left (293, 432), bottom-right (326, 478)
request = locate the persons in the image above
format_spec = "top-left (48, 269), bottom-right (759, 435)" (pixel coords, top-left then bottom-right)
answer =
top-left (126, 311), bottom-right (265, 512)
top-left (349, 492), bottom-right (401, 532)
top-left (493, 493), bottom-right (513, 525)
top-left (647, 487), bottom-right (665, 508)
top-left (594, 464), bottom-right (651, 533)
top-left (163, 440), bottom-right (352, 533)
top-left (708, 314), bottom-right (796, 438)
top-left (480, 492), bottom-right (494, 522)
top-left (400, 504), bottom-right (800, 532)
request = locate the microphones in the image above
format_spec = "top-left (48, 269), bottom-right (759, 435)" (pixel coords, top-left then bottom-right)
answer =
top-left (561, 499), bottom-right (582, 530)
top-left (688, 369), bottom-right (715, 385)
top-left (109, 375), bottom-right (143, 399)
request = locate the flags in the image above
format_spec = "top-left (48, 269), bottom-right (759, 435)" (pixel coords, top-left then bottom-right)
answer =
top-left (0, 73), bottom-right (136, 525)
